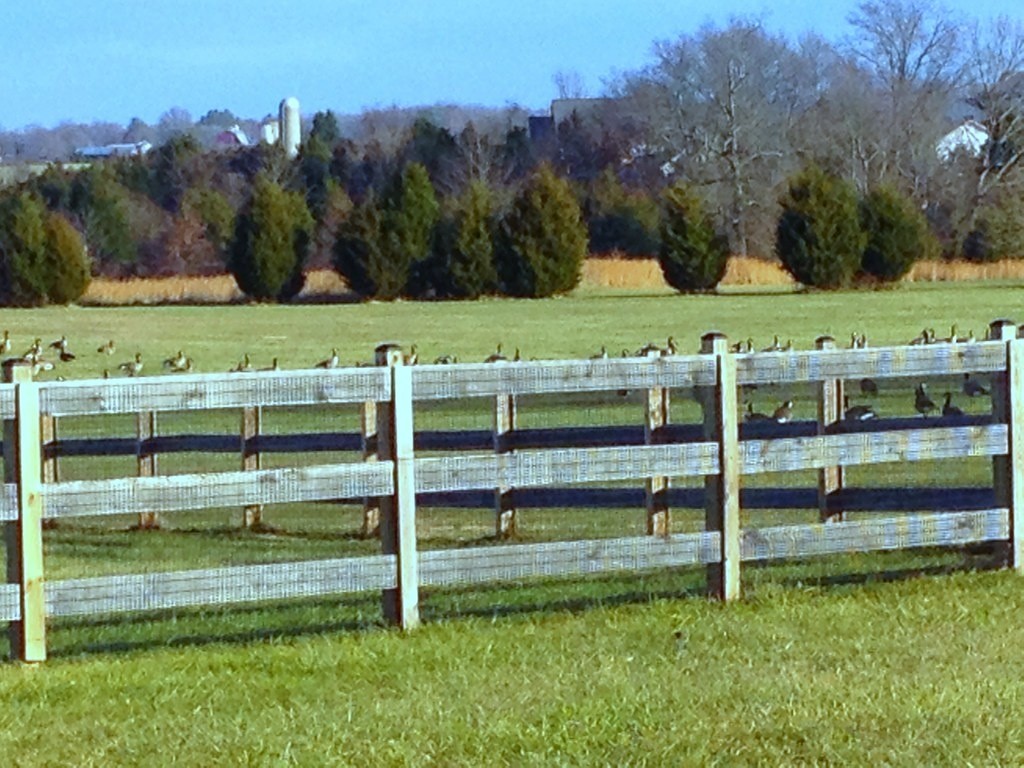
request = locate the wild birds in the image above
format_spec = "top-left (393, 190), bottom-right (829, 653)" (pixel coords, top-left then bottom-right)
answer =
top-left (0, 324), bottom-right (1023, 465)
